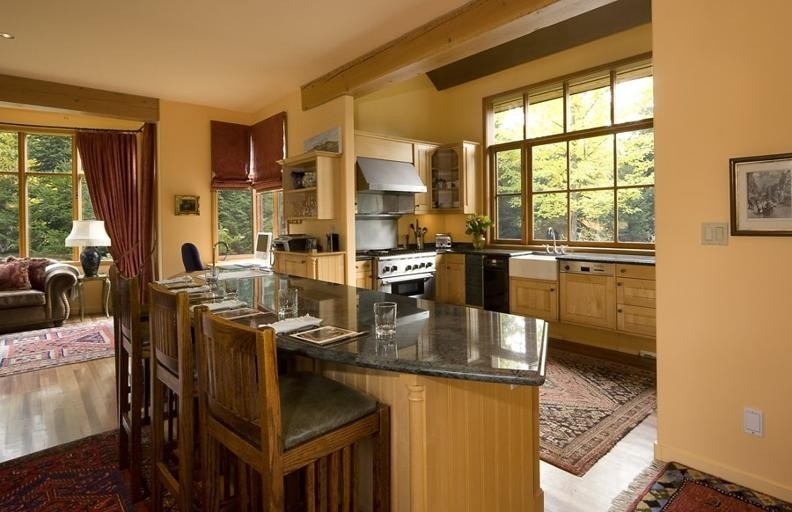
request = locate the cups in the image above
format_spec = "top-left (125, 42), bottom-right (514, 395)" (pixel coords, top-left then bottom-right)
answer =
top-left (205, 270), bottom-right (218, 282)
top-left (375, 302), bottom-right (397, 338)
top-left (308, 239), bottom-right (317, 248)
top-left (277, 288), bottom-right (298, 315)
top-left (225, 278), bottom-right (237, 294)
top-left (376, 339), bottom-right (398, 361)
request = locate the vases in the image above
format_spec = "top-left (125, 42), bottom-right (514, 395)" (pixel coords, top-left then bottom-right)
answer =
top-left (472, 232), bottom-right (486, 249)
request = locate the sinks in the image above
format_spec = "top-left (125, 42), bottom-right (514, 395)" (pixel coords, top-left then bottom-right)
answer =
top-left (198, 269), bottom-right (268, 279)
top-left (508, 253), bottom-right (559, 282)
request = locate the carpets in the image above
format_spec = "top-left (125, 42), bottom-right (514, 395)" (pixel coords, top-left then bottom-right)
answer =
top-left (608, 459), bottom-right (792, 512)
top-left (539, 336), bottom-right (656, 476)
top-left (0, 323), bottom-right (115, 376)
top-left (0, 427), bottom-right (253, 512)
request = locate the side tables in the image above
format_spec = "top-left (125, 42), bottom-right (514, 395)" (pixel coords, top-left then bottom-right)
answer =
top-left (77, 272), bottom-right (112, 322)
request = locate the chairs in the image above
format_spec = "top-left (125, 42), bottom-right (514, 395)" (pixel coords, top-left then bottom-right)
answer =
top-left (146, 282), bottom-right (283, 512)
top-left (194, 304), bottom-right (390, 511)
top-left (181, 243), bottom-right (210, 272)
top-left (118, 273), bottom-right (177, 503)
top-left (0, 258), bottom-right (79, 327)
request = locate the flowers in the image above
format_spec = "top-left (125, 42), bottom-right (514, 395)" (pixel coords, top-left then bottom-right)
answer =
top-left (463, 214), bottom-right (494, 240)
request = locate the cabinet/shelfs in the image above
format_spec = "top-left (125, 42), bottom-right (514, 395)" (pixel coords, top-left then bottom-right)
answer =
top-left (274, 253), bottom-right (345, 284)
top-left (509, 262), bottom-right (656, 370)
top-left (356, 260), bottom-right (373, 291)
top-left (414, 142), bottom-right (479, 214)
top-left (445, 254), bottom-right (466, 306)
top-left (282, 157), bottom-right (338, 222)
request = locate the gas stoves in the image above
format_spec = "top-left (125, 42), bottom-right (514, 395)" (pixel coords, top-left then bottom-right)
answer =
top-left (356, 247), bottom-right (417, 256)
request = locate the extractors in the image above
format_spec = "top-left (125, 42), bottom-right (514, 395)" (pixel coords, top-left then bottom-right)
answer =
top-left (356, 157), bottom-right (428, 195)
top-left (358, 290), bottom-right (430, 353)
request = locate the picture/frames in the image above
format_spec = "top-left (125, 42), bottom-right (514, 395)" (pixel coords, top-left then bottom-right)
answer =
top-left (175, 195), bottom-right (201, 216)
top-left (729, 153), bottom-right (792, 237)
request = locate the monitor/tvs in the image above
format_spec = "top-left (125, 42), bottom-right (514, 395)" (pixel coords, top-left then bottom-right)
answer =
top-left (254, 232), bottom-right (273, 265)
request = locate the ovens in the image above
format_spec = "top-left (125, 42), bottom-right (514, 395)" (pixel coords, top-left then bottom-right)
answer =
top-left (372, 251), bottom-right (437, 301)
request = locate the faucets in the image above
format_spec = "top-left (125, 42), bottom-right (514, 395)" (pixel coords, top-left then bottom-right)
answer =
top-left (546, 226), bottom-right (557, 254)
top-left (211, 240), bottom-right (230, 275)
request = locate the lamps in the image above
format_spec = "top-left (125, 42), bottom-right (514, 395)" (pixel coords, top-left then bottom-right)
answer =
top-left (65, 220), bottom-right (112, 278)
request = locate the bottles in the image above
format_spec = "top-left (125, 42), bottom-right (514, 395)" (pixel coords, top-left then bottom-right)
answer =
top-left (291, 170), bottom-right (315, 188)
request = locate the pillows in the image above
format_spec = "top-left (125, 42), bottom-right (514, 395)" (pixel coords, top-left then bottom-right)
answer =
top-left (0, 255), bottom-right (49, 291)
